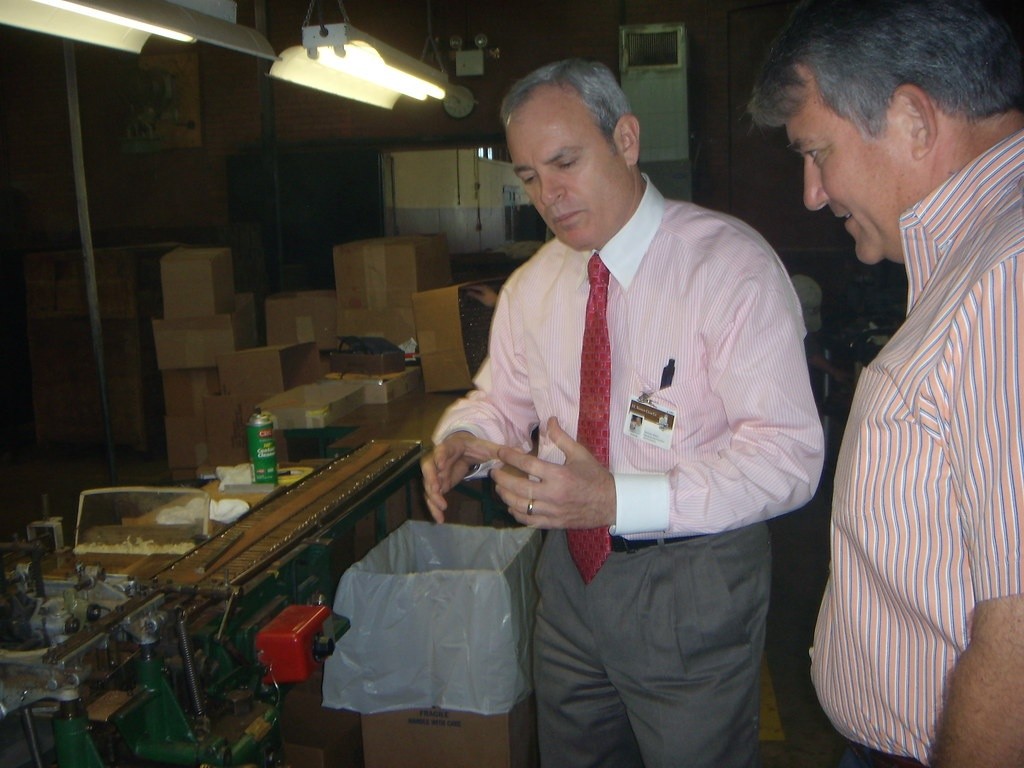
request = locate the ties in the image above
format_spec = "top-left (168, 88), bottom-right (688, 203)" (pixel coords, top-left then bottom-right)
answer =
top-left (565, 252), bottom-right (612, 584)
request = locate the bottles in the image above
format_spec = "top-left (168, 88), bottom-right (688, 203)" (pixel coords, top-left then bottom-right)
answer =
top-left (247, 406), bottom-right (278, 486)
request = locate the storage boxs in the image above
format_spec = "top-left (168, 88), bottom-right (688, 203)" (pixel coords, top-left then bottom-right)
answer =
top-left (27, 232), bottom-right (540, 545)
top-left (255, 516), bottom-right (542, 768)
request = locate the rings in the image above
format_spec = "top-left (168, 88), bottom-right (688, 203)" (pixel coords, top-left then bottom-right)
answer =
top-left (527, 500), bottom-right (533, 515)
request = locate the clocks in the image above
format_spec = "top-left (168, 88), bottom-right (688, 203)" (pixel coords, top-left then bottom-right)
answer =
top-left (442, 85), bottom-right (476, 119)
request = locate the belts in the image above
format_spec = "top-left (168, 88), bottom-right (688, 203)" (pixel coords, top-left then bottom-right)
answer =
top-left (612, 534), bottom-right (704, 553)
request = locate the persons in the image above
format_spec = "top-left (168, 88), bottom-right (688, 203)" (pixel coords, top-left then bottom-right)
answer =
top-left (419, 60), bottom-right (825, 768)
top-left (791, 273), bottom-right (847, 383)
top-left (744, 0), bottom-right (1024, 768)
top-left (631, 416), bottom-right (642, 432)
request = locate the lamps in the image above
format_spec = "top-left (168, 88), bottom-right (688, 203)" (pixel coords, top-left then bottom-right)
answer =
top-left (0, 1), bottom-right (279, 62)
top-left (268, 0), bottom-right (449, 109)
top-left (448, 32), bottom-right (488, 78)
